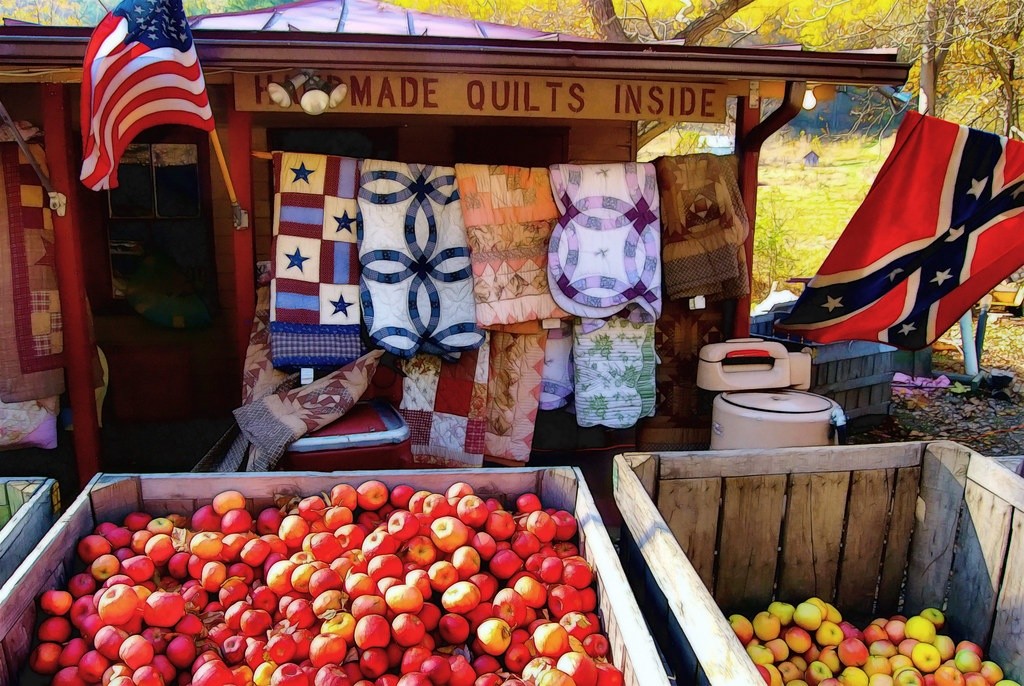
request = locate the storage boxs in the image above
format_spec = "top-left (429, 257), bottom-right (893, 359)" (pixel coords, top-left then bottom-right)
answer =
top-left (0, 435), bottom-right (1024, 686)
top-left (749, 300), bottom-right (898, 442)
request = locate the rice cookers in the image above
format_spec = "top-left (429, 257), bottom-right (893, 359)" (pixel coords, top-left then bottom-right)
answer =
top-left (710, 391), bottom-right (844, 449)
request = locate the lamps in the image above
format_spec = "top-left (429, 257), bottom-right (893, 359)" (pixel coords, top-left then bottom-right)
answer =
top-left (802, 84), bottom-right (818, 110)
top-left (268, 70), bottom-right (348, 116)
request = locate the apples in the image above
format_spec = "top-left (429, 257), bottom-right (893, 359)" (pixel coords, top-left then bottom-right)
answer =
top-left (726, 597), bottom-right (1023, 686)
top-left (28, 481), bottom-right (628, 686)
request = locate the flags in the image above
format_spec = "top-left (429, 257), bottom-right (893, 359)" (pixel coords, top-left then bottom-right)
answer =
top-left (80, 0), bottom-right (215, 192)
top-left (773, 110), bottom-right (1024, 351)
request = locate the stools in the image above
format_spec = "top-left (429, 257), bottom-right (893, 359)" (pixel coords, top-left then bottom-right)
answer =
top-left (280, 400), bottom-right (412, 474)
